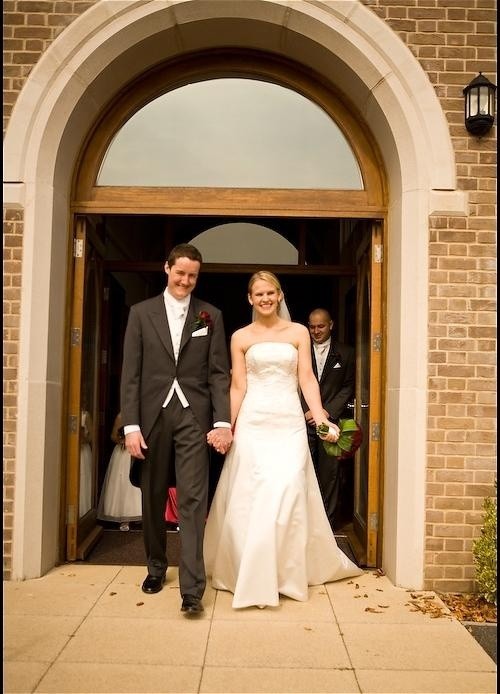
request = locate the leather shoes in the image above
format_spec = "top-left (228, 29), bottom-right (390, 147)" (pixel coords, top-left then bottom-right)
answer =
top-left (142, 575), bottom-right (166, 594)
top-left (180, 595), bottom-right (204, 614)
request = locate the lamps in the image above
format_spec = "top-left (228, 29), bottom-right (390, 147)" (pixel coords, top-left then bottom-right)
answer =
top-left (462, 71), bottom-right (500, 139)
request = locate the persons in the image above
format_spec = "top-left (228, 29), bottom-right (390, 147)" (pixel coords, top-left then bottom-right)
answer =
top-left (118, 242), bottom-right (233, 614)
top-left (110, 412), bottom-right (144, 533)
top-left (299, 308), bottom-right (355, 532)
top-left (206, 269), bottom-right (341, 609)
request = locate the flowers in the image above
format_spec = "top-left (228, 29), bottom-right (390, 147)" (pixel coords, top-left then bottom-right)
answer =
top-left (326, 417), bottom-right (364, 463)
top-left (196, 309), bottom-right (214, 329)
